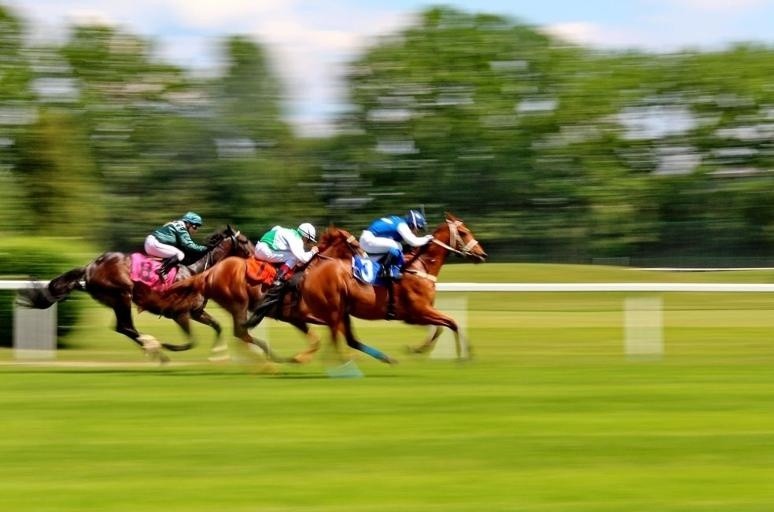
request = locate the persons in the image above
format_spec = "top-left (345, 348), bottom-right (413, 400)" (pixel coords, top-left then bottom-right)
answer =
top-left (357, 208), bottom-right (435, 280)
top-left (143, 209), bottom-right (209, 281)
top-left (253, 221), bottom-right (320, 289)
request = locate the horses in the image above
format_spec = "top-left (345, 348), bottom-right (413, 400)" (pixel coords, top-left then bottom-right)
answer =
top-left (16, 221), bottom-right (256, 357)
top-left (239, 208), bottom-right (490, 366)
top-left (139, 225), bottom-right (402, 370)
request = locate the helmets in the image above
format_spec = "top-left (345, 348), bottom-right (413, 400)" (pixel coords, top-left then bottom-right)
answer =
top-left (298, 222), bottom-right (318, 244)
top-left (411, 209), bottom-right (429, 233)
top-left (181, 211), bottom-right (202, 227)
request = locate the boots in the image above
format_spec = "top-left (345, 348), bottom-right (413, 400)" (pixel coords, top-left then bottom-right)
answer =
top-left (379, 252), bottom-right (395, 277)
top-left (154, 256), bottom-right (179, 280)
top-left (268, 269), bottom-right (286, 292)
